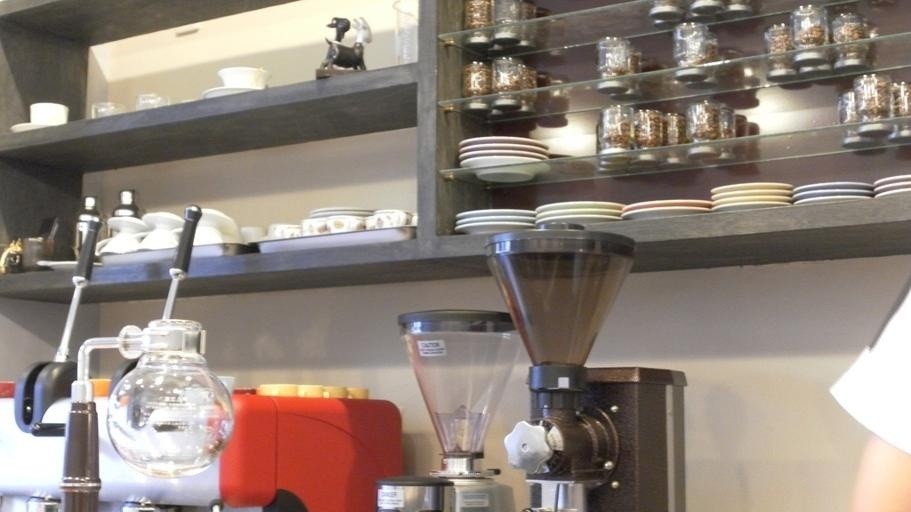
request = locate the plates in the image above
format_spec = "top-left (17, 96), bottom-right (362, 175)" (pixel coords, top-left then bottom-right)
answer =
top-left (36, 259), bottom-right (101, 273)
top-left (9, 121), bottom-right (57, 133)
top-left (874, 174), bottom-right (910, 197)
top-left (202, 86), bottom-right (258, 99)
top-left (457, 134), bottom-right (551, 182)
top-left (792, 182), bottom-right (875, 205)
top-left (454, 209), bottom-right (537, 237)
top-left (536, 200), bottom-right (626, 224)
top-left (710, 182), bottom-right (795, 212)
top-left (311, 206), bottom-right (373, 217)
top-left (621, 198), bottom-right (714, 221)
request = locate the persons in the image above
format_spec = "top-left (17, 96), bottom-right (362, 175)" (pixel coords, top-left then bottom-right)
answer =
top-left (828, 288), bottom-right (909, 512)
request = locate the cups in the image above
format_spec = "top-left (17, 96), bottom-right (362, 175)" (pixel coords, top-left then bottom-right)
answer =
top-left (302, 210), bottom-right (412, 237)
top-left (219, 67), bottom-right (265, 89)
top-left (135, 94), bottom-right (167, 111)
top-left (30, 102), bottom-right (70, 125)
top-left (91, 102), bottom-right (125, 116)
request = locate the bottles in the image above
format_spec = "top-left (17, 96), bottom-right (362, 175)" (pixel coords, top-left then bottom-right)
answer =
top-left (762, 5), bottom-right (876, 77)
top-left (75, 196), bottom-right (103, 258)
top-left (599, 102), bottom-right (750, 167)
top-left (672, 22), bottom-right (720, 88)
top-left (102, 320), bottom-right (234, 480)
top-left (597, 35), bottom-right (644, 97)
top-left (462, 0), bottom-right (572, 118)
top-left (111, 189), bottom-right (139, 236)
top-left (838, 71), bottom-right (911, 147)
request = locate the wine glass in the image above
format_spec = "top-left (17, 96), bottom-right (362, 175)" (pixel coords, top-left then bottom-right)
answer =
top-left (92, 207), bottom-right (235, 256)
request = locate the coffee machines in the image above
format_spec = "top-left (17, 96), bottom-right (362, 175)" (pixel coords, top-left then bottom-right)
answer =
top-left (373, 308), bottom-right (520, 511)
top-left (484, 220), bottom-right (688, 511)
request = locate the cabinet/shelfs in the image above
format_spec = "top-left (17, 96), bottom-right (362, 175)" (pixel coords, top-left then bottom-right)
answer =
top-left (3, 0), bottom-right (911, 305)
